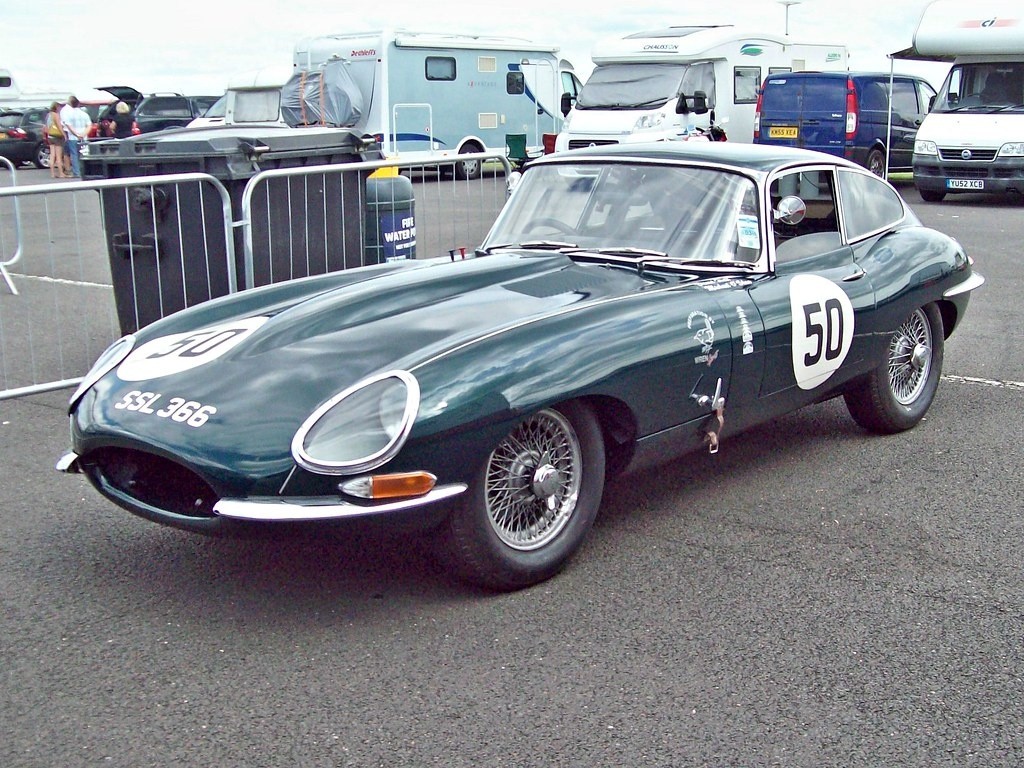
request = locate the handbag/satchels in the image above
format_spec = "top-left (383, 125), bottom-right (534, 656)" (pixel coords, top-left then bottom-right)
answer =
top-left (49, 113), bottom-right (64, 140)
top-left (77, 138), bottom-right (89, 158)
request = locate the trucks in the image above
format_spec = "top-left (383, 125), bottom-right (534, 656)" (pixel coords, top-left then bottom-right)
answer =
top-left (888, 1), bottom-right (1024, 207)
top-left (555, 24), bottom-right (853, 192)
top-left (279, 22), bottom-right (589, 181)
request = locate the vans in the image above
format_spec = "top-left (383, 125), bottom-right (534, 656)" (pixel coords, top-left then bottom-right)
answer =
top-left (752, 70), bottom-right (940, 183)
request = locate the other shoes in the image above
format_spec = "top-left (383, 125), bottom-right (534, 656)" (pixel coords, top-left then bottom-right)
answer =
top-left (59, 172), bottom-right (73, 177)
top-left (51, 173), bottom-right (58, 178)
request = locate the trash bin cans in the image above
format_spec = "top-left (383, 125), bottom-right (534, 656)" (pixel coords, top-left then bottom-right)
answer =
top-left (79, 122), bottom-right (386, 337)
top-left (366, 176), bottom-right (416, 265)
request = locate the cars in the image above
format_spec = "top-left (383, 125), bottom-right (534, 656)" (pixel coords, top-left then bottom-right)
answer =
top-left (54, 137), bottom-right (987, 597)
top-left (0, 69), bottom-right (290, 170)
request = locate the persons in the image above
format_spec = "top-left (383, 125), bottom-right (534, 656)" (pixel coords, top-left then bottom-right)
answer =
top-left (46, 96), bottom-right (144, 178)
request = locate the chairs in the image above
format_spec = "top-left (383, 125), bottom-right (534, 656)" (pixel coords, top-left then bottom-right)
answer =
top-left (861, 81), bottom-right (894, 111)
top-left (504, 133), bottom-right (558, 174)
top-left (979, 73), bottom-right (1008, 105)
